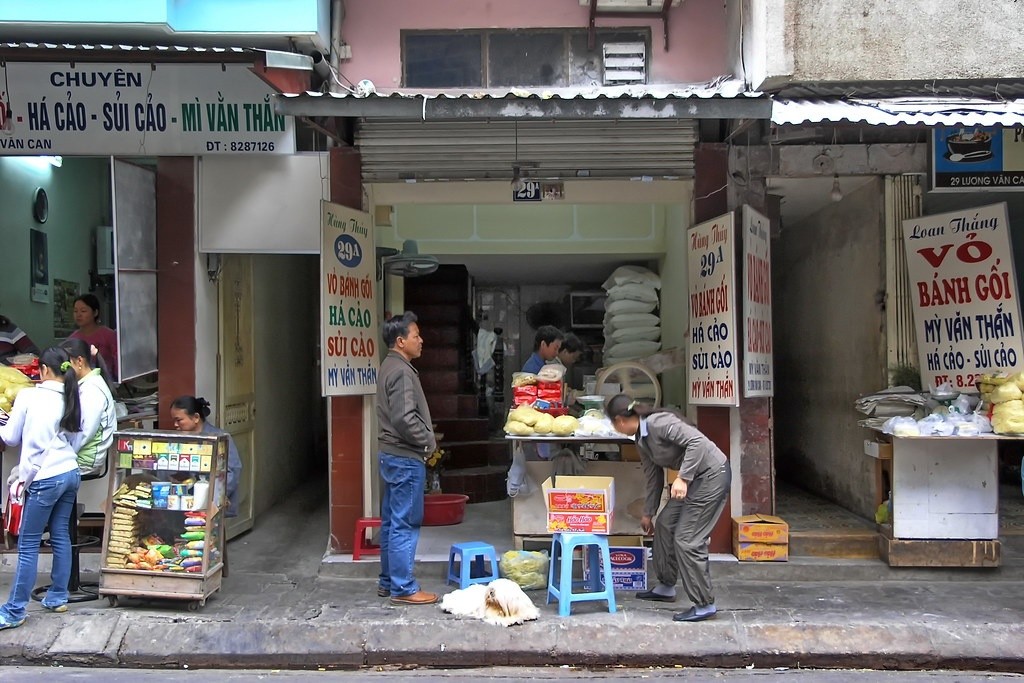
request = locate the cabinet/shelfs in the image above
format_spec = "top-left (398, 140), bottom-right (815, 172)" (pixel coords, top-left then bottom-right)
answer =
top-left (97, 428), bottom-right (232, 607)
top-left (864, 432), bottom-right (1001, 541)
top-left (404, 262), bottom-right (491, 438)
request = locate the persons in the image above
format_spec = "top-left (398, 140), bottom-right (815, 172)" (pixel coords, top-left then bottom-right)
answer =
top-left (171, 394), bottom-right (241, 518)
top-left (6, 338), bottom-right (121, 490)
top-left (607, 393), bottom-right (731, 621)
top-left (376, 311), bottom-right (440, 604)
top-left (0, 346), bottom-right (82, 629)
top-left (66, 294), bottom-right (118, 378)
top-left (522, 326), bottom-right (584, 418)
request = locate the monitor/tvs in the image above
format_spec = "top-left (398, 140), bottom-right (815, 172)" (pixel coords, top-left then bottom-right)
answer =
top-left (96, 226), bottom-right (115, 275)
top-left (570, 290), bottom-right (607, 329)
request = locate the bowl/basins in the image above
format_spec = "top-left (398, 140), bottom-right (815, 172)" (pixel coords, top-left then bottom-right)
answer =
top-left (422, 494), bottom-right (469, 525)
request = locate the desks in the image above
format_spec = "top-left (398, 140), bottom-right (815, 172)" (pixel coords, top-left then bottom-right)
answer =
top-left (502, 433), bottom-right (654, 549)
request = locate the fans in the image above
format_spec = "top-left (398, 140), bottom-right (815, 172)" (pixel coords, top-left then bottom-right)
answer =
top-left (376, 239), bottom-right (439, 282)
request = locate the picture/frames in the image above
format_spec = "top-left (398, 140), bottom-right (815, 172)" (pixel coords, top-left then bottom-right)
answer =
top-left (32, 185), bottom-right (50, 223)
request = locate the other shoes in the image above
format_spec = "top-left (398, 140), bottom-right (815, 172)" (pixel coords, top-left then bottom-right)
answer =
top-left (673, 605), bottom-right (716, 621)
top-left (390, 591), bottom-right (438, 605)
top-left (636, 585), bottom-right (676, 602)
top-left (0, 615), bottom-right (25, 630)
top-left (377, 588), bottom-right (390, 597)
top-left (41, 598), bottom-right (67, 612)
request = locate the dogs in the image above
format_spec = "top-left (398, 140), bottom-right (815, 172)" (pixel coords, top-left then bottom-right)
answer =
top-left (440, 578), bottom-right (541, 627)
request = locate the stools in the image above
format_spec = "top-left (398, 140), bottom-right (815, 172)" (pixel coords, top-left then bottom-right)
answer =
top-left (544, 533), bottom-right (616, 614)
top-left (446, 541), bottom-right (499, 587)
top-left (29, 443), bottom-right (108, 605)
top-left (351, 517), bottom-right (382, 559)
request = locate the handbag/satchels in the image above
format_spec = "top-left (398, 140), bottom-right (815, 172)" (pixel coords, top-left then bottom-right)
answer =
top-left (4, 477), bottom-right (25, 536)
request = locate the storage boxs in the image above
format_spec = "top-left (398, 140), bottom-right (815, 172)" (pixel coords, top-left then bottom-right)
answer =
top-left (731, 513), bottom-right (789, 543)
top-left (118, 437), bottom-right (227, 473)
top-left (542, 474), bottom-right (615, 534)
top-left (581, 534), bottom-right (650, 591)
top-left (732, 536), bottom-right (790, 563)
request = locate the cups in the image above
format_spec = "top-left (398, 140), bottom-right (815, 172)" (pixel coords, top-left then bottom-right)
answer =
top-left (150, 482), bottom-right (172, 508)
top-left (167, 495), bottom-right (181, 510)
top-left (181, 496), bottom-right (194, 510)
top-left (171, 484), bottom-right (188, 496)
